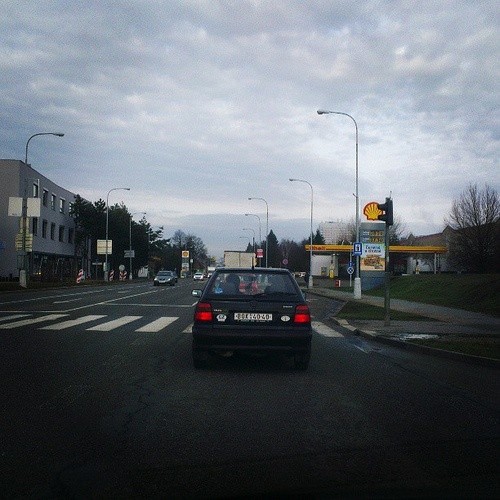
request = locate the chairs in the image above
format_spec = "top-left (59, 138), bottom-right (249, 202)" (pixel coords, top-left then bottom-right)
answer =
top-left (218, 281), bottom-right (236, 296)
top-left (269, 280), bottom-right (288, 293)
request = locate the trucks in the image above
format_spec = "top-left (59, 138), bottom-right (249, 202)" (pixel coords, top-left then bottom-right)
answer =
top-left (224, 250), bottom-right (256, 278)
top-left (208, 266), bottom-right (216, 278)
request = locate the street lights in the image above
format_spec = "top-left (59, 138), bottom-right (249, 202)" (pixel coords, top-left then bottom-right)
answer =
top-left (242, 228), bottom-right (255, 251)
top-left (20, 131), bottom-right (64, 286)
top-left (248, 197), bottom-right (269, 283)
top-left (317, 108), bottom-right (362, 300)
top-left (329, 221), bottom-right (352, 287)
top-left (241, 236), bottom-right (250, 250)
top-left (245, 214), bottom-right (262, 284)
top-left (105, 187), bottom-right (131, 282)
top-left (129, 212), bottom-right (146, 280)
top-left (147, 225), bottom-right (163, 281)
top-left (289, 178), bottom-right (313, 288)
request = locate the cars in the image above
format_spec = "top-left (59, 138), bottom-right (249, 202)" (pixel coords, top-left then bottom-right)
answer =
top-left (193, 273), bottom-right (204, 281)
top-left (299, 272), bottom-right (305, 278)
top-left (236, 273), bottom-right (257, 295)
top-left (191, 263), bottom-right (313, 371)
top-left (153, 271), bottom-right (178, 287)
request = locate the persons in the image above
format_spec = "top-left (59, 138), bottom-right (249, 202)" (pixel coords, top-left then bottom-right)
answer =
top-left (243, 272), bottom-right (258, 296)
top-left (224, 273), bottom-right (244, 295)
top-left (264, 275), bottom-right (291, 294)
top-left (416, 261), bottom-right (420, 274)
top-left (304, 271), bottom-right (310, 288)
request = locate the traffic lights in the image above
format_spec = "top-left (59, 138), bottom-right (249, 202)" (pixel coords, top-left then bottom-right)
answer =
top-left (377, 198), bottom-right (394, 224)
